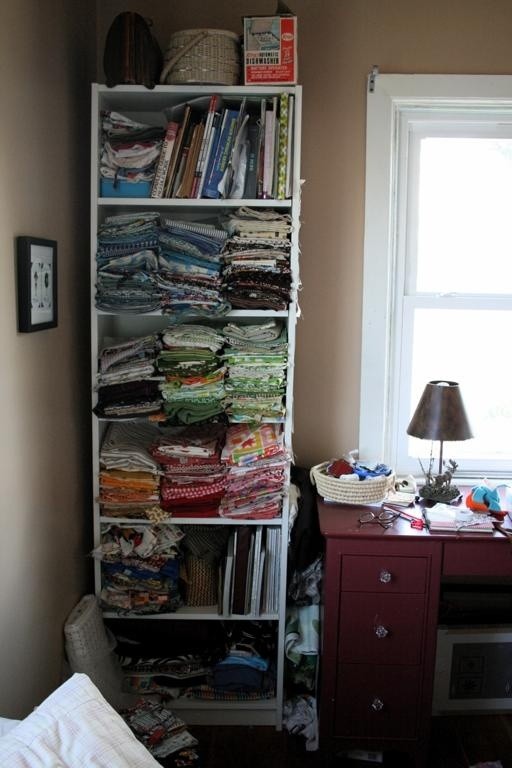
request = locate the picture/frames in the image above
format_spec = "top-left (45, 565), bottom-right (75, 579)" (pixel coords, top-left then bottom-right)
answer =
top-left (14, 237), bottom-right (61, 335)
top-left (241, 9), bottom-right (300, 89)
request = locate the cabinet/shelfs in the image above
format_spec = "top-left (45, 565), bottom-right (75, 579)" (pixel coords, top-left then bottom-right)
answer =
top-left (86, 80), bottom-right (303, 731)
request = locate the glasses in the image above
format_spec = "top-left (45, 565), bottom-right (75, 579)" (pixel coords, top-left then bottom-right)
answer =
top-left (359, 511), bottom-right (400, 529)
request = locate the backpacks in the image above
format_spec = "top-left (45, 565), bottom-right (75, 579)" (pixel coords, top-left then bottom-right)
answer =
top-left (103, 11), bottom-right (164, 89)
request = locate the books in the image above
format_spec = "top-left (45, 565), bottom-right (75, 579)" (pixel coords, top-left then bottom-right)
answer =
top-left (146, 93), bottom-right (296, 200)
top-left (216, 524), bottom-right (282, 620)
top-left (424, 501), bottom-right (494, 533)
top-left (239, 14), bottom-right (301, 89)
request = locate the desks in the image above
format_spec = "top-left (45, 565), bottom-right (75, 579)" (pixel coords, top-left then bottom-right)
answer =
top-left (312, 488), bottom-right (512, 754)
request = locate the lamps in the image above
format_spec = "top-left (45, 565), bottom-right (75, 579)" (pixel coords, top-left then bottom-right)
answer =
top-left (404, 377), bottom-right (476, 504)
